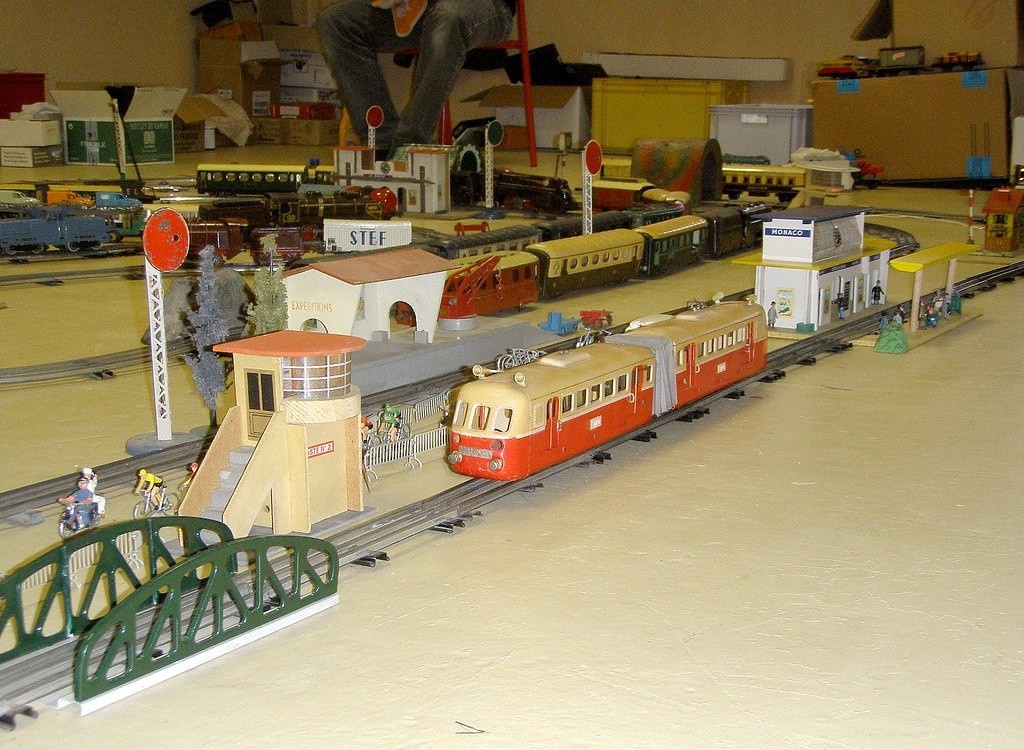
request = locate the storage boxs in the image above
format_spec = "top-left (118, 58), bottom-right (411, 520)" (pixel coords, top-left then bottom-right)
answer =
top-left (707, 0), bottom-right (1024, 182)
top-left (461, 80), bottom-right (592, 150)
top-left (592, 77), bottom-right (741, 156)
top-left (0, 0), bottom-right (347, 168)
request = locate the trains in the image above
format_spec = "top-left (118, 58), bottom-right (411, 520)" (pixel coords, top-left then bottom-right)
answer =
top-left (814, 45), bottom-right (986, 78)
top-left (395, 201), bottom-right (772, 328)
top-left (193, 163), bottom-right (574, 215)
top-left (411, 174), bottom-right (693, 261)
top-left (444, 291), bottom-right (769, 481)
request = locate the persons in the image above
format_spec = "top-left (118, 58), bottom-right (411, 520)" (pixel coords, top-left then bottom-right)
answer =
top-left (918, 293), bottom-right (952, 329)
top-left (768, 301), bottom-right (778, 328)
top-left (377, 413), bottom-right (400, 442)
top-left (58, 478), bottom-right (94, 530)
top-left (183, 463), bottom-right (199, 486)
top-left (361, 416), bottom-right (374, 443)
top-left (898, 304), bottom-right (906, 324)
top-left (834, 294), bottom-right (845, 319)
top-left (135, 469), bottom-right (165, 512)
top-left (893, 308), bottom-right (902, 327)
top-left (871, 280), bottom-right (884, 305)
top-left (82, 468), bottom-right (106, 519)
top-left (384, 403), bottom-right (402, 418)
top-left (878, 311), bottom-right (890, 332)
top-left (312, 0), bottom-right (518, 161)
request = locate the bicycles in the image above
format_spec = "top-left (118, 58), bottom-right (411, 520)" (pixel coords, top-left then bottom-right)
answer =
top-left (133, 484), bottom-right (182, 520)
top-left (359, 431), bottom-right (384, 458)
top-left (375, 418), bottom-right (413, 452)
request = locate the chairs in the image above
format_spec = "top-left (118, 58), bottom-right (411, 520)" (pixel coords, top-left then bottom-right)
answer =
top-left (372, 1), bottom-right (537, 167)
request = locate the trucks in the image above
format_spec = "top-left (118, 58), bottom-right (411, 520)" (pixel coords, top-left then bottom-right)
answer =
top-left (1, 181), bottom-right (152, 257)
top-left (177, 185), bottom-right (396, 266)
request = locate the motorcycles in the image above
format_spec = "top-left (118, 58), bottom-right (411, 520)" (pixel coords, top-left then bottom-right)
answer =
top-left (56, 499), bottom-right (102, 540)
